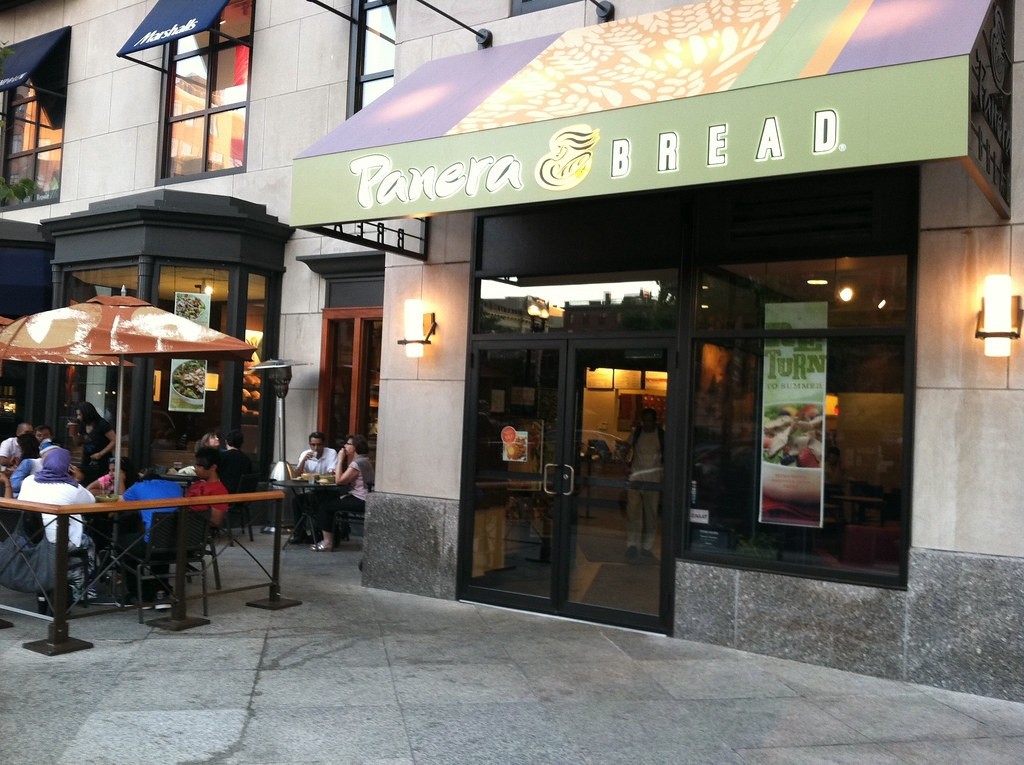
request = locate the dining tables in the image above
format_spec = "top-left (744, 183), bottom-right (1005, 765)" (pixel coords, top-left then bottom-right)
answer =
top-left (272, 479), bottom-right (345, 551)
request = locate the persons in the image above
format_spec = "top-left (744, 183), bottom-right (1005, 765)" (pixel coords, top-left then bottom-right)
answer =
top-left (290, 432), bottom-right (338, 542)
top-left (1, 402), bottom-right (254, 608)
top-left (311, 435), bottom-right (375, 552)
top-left (618, 410), bottom-right (665, 556)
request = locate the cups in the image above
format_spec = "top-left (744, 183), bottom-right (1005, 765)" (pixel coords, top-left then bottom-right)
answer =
top-left (66, 422), bottom-right (78, 436)
top-left (174, 461), bottom-right (183, 471)
top-left (308, 477), bottom-right (314, 484)
top-left (309, 450), bottom-right (317, 460)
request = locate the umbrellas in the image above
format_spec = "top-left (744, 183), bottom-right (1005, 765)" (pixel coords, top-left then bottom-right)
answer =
top-left (0, 296), bottom-right (258, 494)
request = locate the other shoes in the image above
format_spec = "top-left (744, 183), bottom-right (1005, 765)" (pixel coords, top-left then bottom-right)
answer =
top-left (155, 590), bottom-right (176, 609)
top-left (641, 547), bottom-right (652, 557)
top-left (290, 529), bottom-right (306, 542)
top-left (100, 569), bottom-right (123, 584)
top-left (88, 592), bottom-right (117, 606)
top-left (310, 543), bottom-right (333, 552)
top-left (307, 531), bottom-right (324, 544)
top-left (625, 546), bottom-right (639, 558)
top-left (115, 593), bottom-right (153, 610)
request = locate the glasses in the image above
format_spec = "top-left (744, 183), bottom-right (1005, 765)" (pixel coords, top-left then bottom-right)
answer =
top-left (344, 442), bottom-right (353, 446)
top-left (193, 463), bottom-right (206, 472)
top-left (310, 443), bottom-right (323, 446)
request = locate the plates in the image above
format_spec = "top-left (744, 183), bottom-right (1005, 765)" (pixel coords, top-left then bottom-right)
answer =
top-left (290, 476), bottom-right (304, 481)
top-left (316, 480), bottom-right (333, 484)
top-left (96, 495), bottom-right (119, 503)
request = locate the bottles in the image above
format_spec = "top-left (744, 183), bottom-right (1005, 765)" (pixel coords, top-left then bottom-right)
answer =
top-left (691, 481), bottom-right (697, 503)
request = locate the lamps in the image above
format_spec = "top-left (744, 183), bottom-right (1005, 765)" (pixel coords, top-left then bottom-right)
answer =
top-left (975, 275), bottom-right (1024, 358)
top-left (397, 299), bottom-right (437, 358)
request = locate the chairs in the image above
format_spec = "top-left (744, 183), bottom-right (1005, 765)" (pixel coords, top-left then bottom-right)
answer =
top-left (0, 471), bottom-right (374, 621)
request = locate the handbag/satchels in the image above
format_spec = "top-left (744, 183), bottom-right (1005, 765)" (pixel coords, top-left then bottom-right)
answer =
top-left (0, 510), bottom-right (56, 593)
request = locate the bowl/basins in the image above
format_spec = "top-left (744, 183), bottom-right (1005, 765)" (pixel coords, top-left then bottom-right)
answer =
top-left (154, 464), bottom-right (166, 474)
top-left (320, 475), bottom-right (335, 481)
top-left (763, 401), bottom-right (823, 504)
top-left (301, 473), bottom-right (319, 480)
top-left (171, 360), bottom-right (206, 405)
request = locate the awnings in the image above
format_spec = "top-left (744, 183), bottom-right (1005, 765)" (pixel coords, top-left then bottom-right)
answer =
top-left (0, 26), bottom-right (72, 96)
top-left (117, 0), bottom-right (252, 71)
top-left (287, 0), bottom-right (1015, 229)
